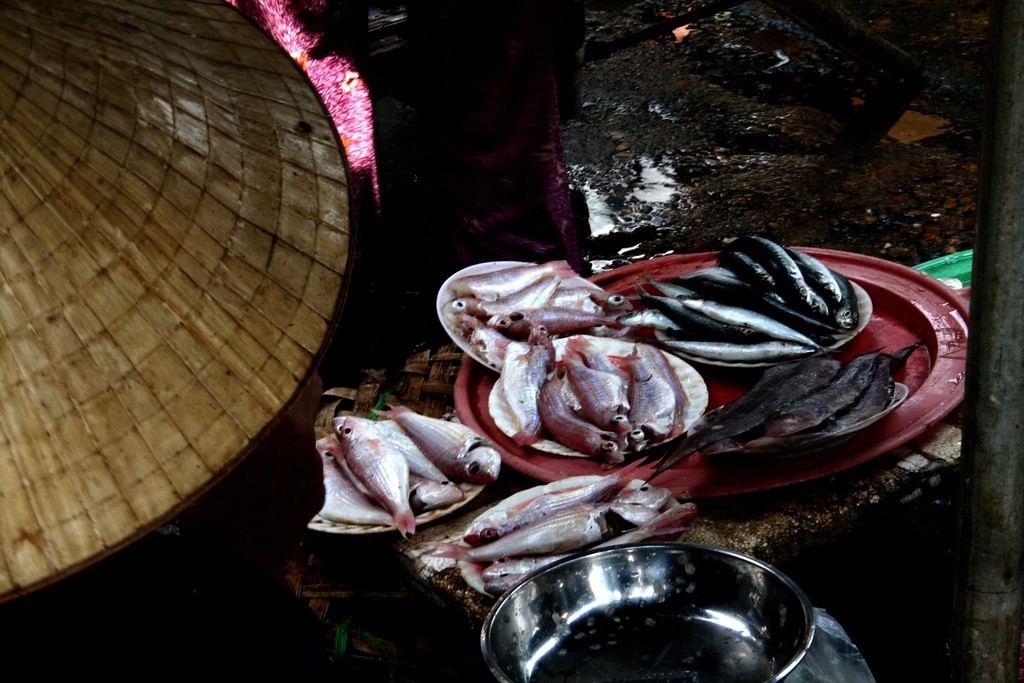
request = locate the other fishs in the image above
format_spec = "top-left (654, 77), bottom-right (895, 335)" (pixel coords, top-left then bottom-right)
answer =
top-left (445, 260), bottom-right (691, 470)
top-left (400, 463), bottom-right (700, 597)
top-left (315, 402), bottom-right (502, 539)
top-left (635, 339), bottom-right (924, 481)
top-left (615, 233), bottom-right (861, 361)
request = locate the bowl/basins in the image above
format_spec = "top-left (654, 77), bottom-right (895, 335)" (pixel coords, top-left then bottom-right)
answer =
top-left (477, 540), bottom-right (819, 683)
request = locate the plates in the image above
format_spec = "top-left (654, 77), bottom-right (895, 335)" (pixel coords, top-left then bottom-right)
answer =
top-left (488, 343), bottom-right (708, 456)
top-left (436, 261), bottom-right (605, 374)
top-left (653, 279), bottom-right (873, 368)
top-left (803, 380), bottom-right (909, 442)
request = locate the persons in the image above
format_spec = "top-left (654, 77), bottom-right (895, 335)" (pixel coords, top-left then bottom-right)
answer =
top-left (0, 0), bottom-right (329, 683)
